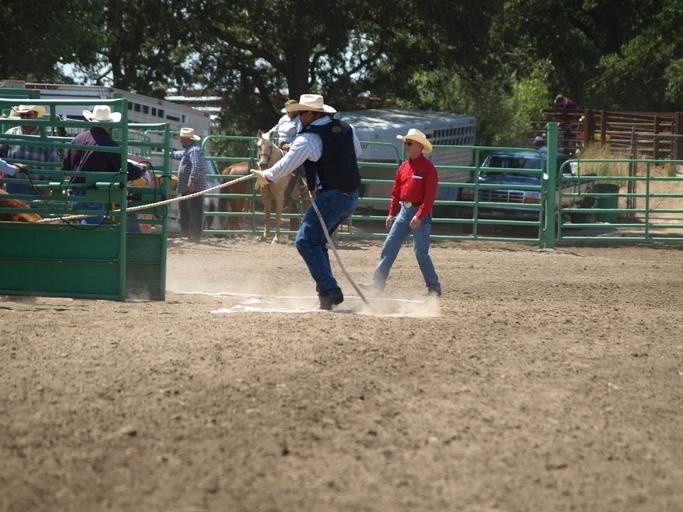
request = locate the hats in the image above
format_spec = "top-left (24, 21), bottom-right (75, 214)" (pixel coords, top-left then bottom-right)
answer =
top-left (280, 94), bottom-right (337, 114)
top-left (175, 128), bottom-right (201, 141)
top-left (396, 129), bottom-right (433, 154)
top-left (8, 105), bottom-right (46, 120)
top-left (82, 105), bottom-right (122, 122)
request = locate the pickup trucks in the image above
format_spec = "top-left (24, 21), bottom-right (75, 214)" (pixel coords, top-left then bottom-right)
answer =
top-left (458, 149), bottom-right (598, 227)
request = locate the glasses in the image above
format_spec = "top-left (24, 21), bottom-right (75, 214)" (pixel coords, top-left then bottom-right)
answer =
top-left (403, 142), bottom-right (413, 145)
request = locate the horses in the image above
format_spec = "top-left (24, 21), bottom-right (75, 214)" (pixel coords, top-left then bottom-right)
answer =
top-left (256, 128), bottom-right (309, 246)
top-left (217, 159), bottom-right (312, 241)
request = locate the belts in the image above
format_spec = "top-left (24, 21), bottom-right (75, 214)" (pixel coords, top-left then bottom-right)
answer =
top-left (399, 201), bottom-right (419, 208)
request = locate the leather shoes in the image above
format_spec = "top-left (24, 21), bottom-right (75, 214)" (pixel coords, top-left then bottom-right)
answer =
top-left (424, 287), bottom-right (440, 296)
top-left (319, 293), bottom-right (343, 309)
top-left (358, 283), bottom-right (383, 295)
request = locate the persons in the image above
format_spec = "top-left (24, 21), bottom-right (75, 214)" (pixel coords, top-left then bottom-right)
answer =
top-left (553, 94), bottom-right (579, 156)
top-left (60, 104), bottom-right (152, 233)
top-left (0, 158), bottom-right (25, 176)
top-left (265, 99), bottom-right (304, 210)
top-left (154, 127), bottom-right (204, 244)
top-left (0, 105), bottom-right (61, 194)
top-left (357, 127), bottom-right (443, 301)
top-left (249, 94), bottom-right (362, 312)
